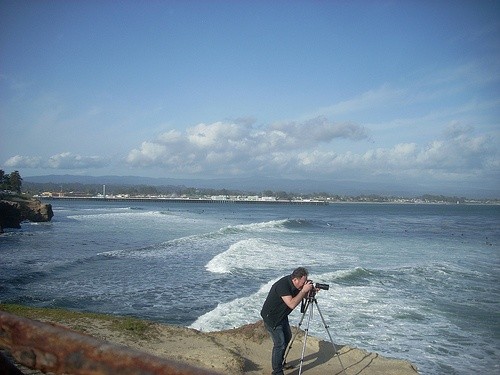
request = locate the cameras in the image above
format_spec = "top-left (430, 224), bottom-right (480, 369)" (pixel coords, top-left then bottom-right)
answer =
top-left (305, 280), bottom-right (329, 298)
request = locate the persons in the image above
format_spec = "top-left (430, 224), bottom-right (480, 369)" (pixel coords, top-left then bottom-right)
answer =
top-left (260, 267), bottom-right (315, 375)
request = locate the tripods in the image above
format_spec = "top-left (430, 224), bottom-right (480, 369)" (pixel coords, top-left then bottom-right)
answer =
top-left (282, 298), bottom-right (348, 375)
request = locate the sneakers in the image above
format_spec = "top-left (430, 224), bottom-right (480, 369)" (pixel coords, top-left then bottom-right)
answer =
top-left (282, 363), bottom-right (294, 370)
